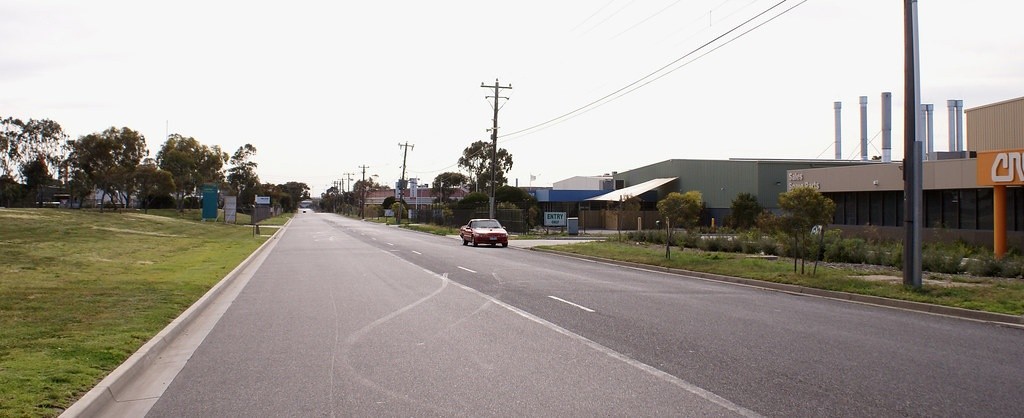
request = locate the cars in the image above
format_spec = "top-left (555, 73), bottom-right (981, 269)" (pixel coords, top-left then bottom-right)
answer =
top-left (459, 219), bottom-right (509, 247)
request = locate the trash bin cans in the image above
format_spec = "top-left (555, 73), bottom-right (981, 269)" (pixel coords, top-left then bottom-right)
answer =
top-left (567, 218), bottom-right (578, 236)
top-left (256, 224), bottom-right (261, 234)
